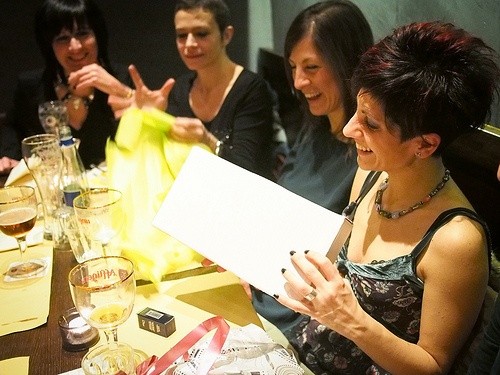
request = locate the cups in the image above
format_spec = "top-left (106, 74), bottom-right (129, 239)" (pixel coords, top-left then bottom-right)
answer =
top-left (58, 307), bottom-right (100, 351)
top-left (22, 134), bottom-right (61, 240)
top-left (56, 208), bottom-right (85, 262)
top-left (81, 341), bottom-right (135, 375)
top-left (38, 101), bottom-right (68, 134)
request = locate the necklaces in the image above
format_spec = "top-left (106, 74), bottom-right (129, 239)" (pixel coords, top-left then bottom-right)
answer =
top-left (375, 166), bottom-right (450, 218)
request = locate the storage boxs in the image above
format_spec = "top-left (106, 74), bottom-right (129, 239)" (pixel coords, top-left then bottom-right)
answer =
top-left (136, 307), bottom-right (177, 339)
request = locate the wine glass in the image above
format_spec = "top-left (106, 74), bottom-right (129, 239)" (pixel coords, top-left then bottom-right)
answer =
top-left (0, 185), bottom-right (46, 278)
top-left (68, 256), bottom-right (142, 373)
top-left (73, 186), bottom-right (124, 257)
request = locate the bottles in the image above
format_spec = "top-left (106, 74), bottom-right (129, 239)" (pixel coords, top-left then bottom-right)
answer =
top-left (57, 126), bottom-right (90, 211)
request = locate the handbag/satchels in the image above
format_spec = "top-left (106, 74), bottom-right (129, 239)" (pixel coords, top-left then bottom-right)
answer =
top-left (118, 317), bottom-right (306, 375)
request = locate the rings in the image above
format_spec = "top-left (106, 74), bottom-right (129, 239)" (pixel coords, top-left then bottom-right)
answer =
top-left (304, 287), bottom-right (318, 301)
top-left (127, 87), bottom-right (133, 99)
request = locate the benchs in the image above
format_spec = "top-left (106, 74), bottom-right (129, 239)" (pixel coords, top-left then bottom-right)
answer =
top-left (258, 47), bottom-right (500, 375)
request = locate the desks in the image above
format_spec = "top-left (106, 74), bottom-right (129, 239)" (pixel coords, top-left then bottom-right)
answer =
top-left (0, 144), bottom-right (264, 375)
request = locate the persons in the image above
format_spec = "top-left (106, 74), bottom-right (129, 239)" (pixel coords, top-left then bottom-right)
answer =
top-left (0, 0), bottom-right (500, 375)
